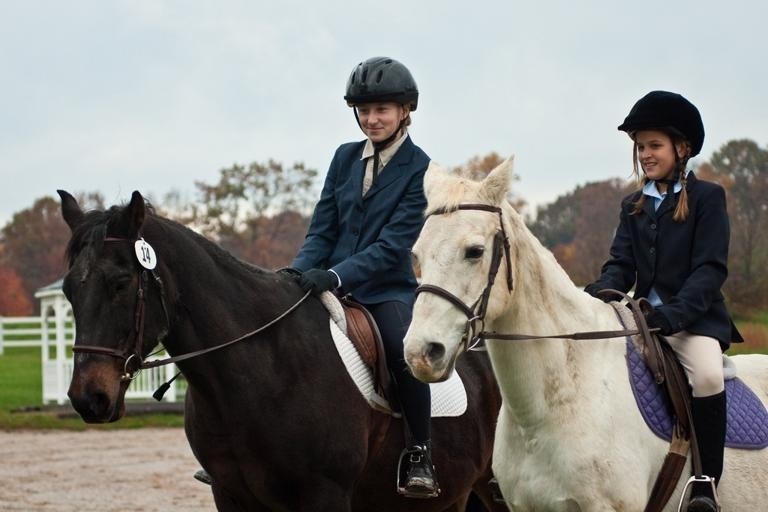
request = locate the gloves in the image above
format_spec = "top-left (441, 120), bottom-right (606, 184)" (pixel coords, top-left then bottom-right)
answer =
top-left (299, 268), bottom-right (339, 298)
top-left (645, 309), bottom-right (672, 336)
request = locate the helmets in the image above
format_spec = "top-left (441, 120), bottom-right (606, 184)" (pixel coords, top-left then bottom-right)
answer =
top-left (344, 56), bottom-right (418, 111)
top-left (618, 90), bottom-right (705, 158)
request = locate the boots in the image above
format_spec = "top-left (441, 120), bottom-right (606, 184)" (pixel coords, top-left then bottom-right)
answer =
top-left (687, 389), bottom-right (727, 511)
top-left (405, 439), bottom-right (435, 491)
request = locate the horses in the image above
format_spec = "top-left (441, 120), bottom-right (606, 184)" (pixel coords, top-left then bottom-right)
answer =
top-left (402, 154), bottom-right (768, 512)
top-left (55, 189), bottom-right (510, 512)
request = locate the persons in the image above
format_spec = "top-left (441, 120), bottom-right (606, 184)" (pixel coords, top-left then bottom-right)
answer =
top-left (192, 56), bottom-right (438, 490)
top-left (582, 90), bottom-right (744, 512)
